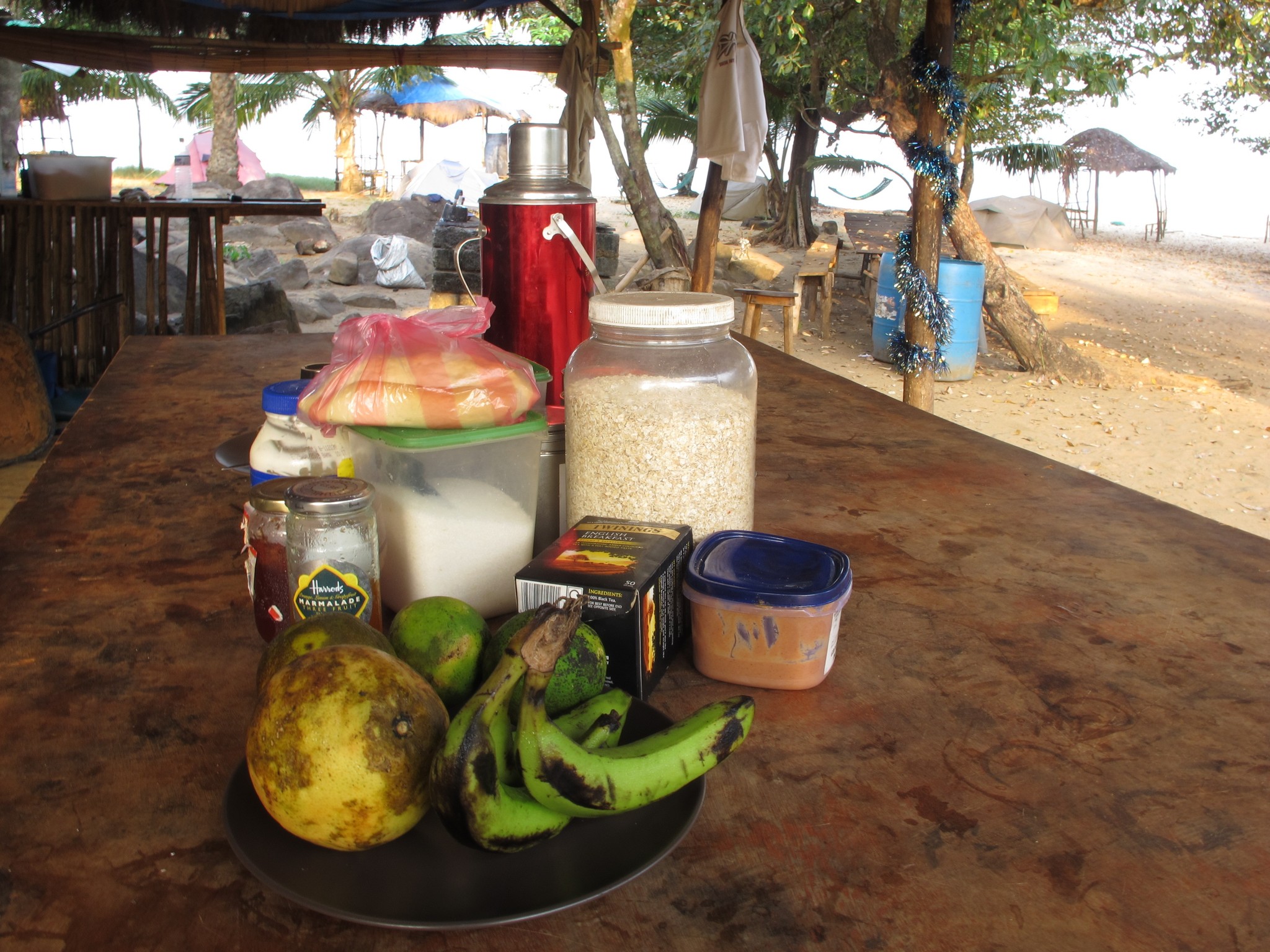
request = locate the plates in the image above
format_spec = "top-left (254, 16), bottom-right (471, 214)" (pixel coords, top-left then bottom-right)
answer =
top-left (219, 688), bottom-right (704, 931)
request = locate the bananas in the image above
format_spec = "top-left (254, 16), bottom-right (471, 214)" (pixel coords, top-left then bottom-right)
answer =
top-left (430, 591), bottom-right (754, 853)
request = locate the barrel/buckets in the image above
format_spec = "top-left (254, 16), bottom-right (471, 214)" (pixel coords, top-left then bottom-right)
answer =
top-left (871, 251), bottom-right (902, 364)
top-left (894, 258), bottom-right (985, 382)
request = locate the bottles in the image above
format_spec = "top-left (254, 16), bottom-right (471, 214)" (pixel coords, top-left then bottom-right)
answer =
top-left (564, 291), bottom-right (758, 543)
top-left (174, 138), bottom-right (193, 203)
top-left (248, 380), bottom-right (350, 482)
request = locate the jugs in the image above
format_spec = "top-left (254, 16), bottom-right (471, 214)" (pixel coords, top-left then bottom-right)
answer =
top-left (452, 121), bottom-right (607, 455)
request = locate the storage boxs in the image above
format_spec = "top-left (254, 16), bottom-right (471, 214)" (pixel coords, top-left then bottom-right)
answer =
top-left (510, 515), bottom-right (691, 703)
top-left (346, 405), bottom-right (550, 617)
top-left (18, 155), bottom-right (117, 200)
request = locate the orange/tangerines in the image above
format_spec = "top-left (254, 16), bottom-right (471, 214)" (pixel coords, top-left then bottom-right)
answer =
top-left (484, 606), bottom-right (607, 719)
top-left (389, 597), bottom-right (496, 705)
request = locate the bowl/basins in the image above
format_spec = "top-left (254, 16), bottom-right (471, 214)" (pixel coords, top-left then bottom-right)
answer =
top-left (681, 530), bottom-right (855, 690)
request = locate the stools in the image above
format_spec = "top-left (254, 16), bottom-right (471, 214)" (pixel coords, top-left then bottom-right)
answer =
top-left (733, 287), bottom-right (800, 356)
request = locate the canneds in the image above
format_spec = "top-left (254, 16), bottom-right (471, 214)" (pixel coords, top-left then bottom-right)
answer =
top-left (285, 476), bottom-right (382, 633)
top-left (241, 478), bottom-right (308, 641)
top-left (532, 405), bottom-right (568, 559)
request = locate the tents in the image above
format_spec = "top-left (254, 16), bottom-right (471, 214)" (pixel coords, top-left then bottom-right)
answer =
top-left (967, 194), bottom-right (1080, 251)
top-left (688, 173), bottom-right (770, 222)
top-left (154, 124), bottom-right (266, 187)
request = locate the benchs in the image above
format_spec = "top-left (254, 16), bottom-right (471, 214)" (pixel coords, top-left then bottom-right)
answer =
top-left (790, 232), bottom-right (840, 339)
top-left (1005, 266), bottom-right (1059, 313)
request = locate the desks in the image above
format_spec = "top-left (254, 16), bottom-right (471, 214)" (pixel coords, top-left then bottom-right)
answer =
top-left (0, 315), bottom-right (1270, 952)
top-left (0, 193), bottom-right (337, 399)
top-left (843, 206), bottom-right (956, 308)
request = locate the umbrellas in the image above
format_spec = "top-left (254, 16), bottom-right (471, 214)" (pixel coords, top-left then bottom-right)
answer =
top-left (351, 65), bottom-right (517, 165)
top-left (1056, 127), bottom-right (1177, 235)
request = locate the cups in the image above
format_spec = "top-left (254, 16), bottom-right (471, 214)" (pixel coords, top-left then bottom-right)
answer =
top-left (300, 361), bottom-right (328, 380)
